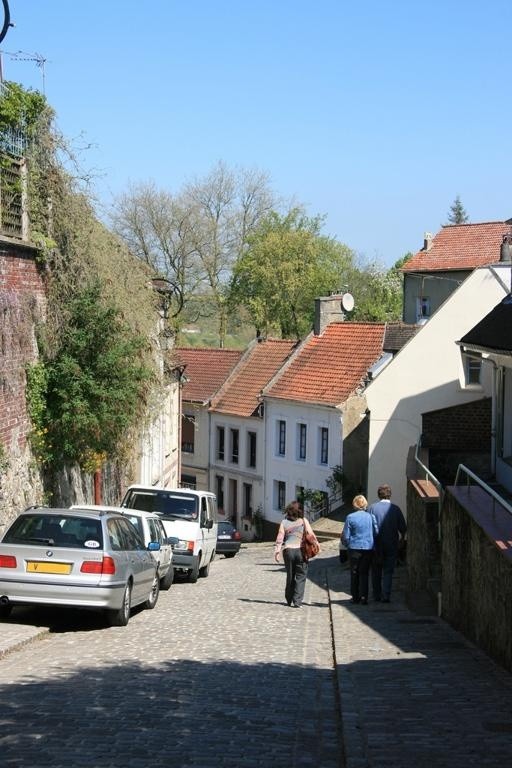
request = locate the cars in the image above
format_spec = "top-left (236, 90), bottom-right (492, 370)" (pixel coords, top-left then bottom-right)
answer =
top-left (0, 484), bottom-right (243, 625)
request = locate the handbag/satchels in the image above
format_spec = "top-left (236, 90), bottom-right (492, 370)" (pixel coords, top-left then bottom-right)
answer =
top-left (301, 517), bottom-right (319, 562)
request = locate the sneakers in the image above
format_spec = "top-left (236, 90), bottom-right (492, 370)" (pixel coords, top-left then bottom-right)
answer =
top-left (287, 598), bottom-right (391, 608)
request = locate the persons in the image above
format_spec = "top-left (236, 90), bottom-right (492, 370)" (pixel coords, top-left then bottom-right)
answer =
top-left (274, 500), bottom-right (321, 608)
top-left (340, 494), bottom-right (380, 605)
top-left (367, 484), bottom-right (408, 604)
